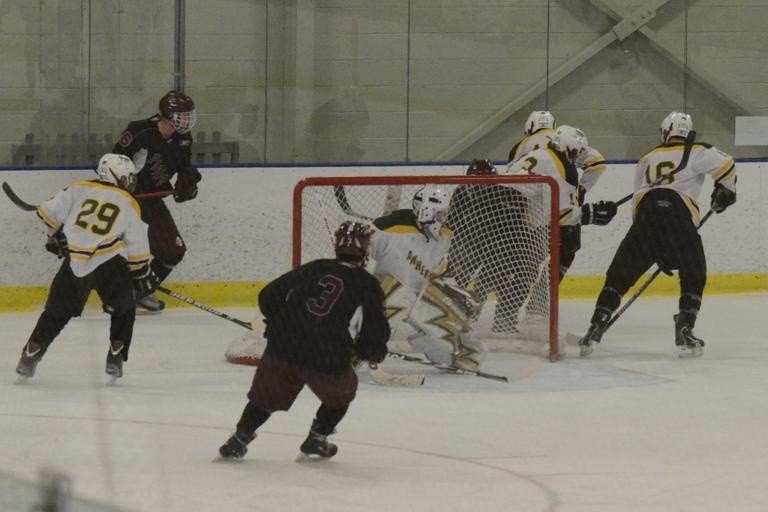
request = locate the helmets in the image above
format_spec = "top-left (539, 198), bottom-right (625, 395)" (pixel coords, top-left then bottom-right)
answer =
top-left (96, 150), bottom-right (138, 190)
top-left (412, 188), bottom-right (448, 225)
top-left (468, 158), bottom-right (495, 175)
top-left (159, 85), bottom-right (199, 134)
top-left (552, 125), bottom-right (589, 159)
top-left (524, 110), bottom-right (556, 137)
top-left (658, 110), bottom-right (696, 144)
top-left (334, 220), bottom-right (376, 264)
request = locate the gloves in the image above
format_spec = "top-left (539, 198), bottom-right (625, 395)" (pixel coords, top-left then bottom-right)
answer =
top-left (134, 272), bottom-right (160, 294)
top-left (581, 199), bottom-right (618, 226)
top-left (656, 260), bottom-right (681, 276)
top-left (173, 166), bottom-right (201, 203)
top-left (711, 184), bottom-right (738, 216)
top-left (44, 235), bottom-right (68, 259)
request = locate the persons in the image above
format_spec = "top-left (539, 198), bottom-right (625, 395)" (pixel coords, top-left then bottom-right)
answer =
top-left (579, 110), bottom-right (739, 360)
top-left (446, 159), bottom-right (541, 337)
top-left (365, 188), bottom-right (482, 374)
top-left (467, 126), bottom-right (618, 337)
top-left (98, 90), bottom-right (203, 310)
top-left (473, 111), bottom-right (608, 331)
top-left (219, 221), bottom-right (391, 462)
top-left (15, 153), bottom-right (160, 386)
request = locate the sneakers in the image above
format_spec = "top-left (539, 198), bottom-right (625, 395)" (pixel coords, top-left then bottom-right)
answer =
top-left (138, 295), bottom-right (166, 312)
top-left (674, 331), bottom-right (704, 348)
top-left (218, 443), bottom-right (248, 458)
top-left (298, 438), bottom-right (339, 459)
top-left (578, 333), bottom-right (601, 346)
top-left (16, 362), bottom-right (35, 379)
top-left (105, 357), bottom-right (124, 379)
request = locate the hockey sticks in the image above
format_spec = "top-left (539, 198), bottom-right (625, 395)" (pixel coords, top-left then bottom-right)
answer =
top-left (155, 284), bottom-right (266, 335)
top-left (388, 351), bottom-right (509, 384)
top-left (2, 181), bottom-right (175, 210)
top-left (367, 258), bottom-right (431, 388)
top-left (565, 208), bottom-right (716, 347)
top-left (333, 184), bottom-right (375, 222)
top-left (613, 128), bottom-right (698, 208)
